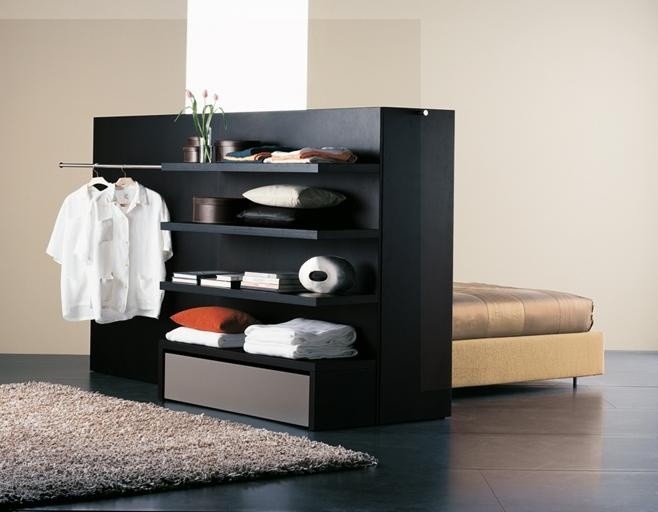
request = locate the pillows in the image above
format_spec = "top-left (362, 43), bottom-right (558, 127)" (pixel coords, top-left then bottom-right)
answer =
top-left (169, 306), bottom-right (255, 336)
top-left (240, 184), bottom-right (348, 212)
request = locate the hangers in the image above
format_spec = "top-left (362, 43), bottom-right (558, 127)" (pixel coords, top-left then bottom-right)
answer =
top-left (110, 166), bottom-right (151, 195)
top-left (86, 163), bottom-right (113, 189)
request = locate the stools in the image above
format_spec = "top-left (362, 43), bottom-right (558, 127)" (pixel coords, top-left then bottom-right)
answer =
top-left (452, 282), bottom-right (603, 390)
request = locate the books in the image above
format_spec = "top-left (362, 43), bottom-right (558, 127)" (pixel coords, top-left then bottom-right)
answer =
top-left (172, 269), bottom-right (307, 294)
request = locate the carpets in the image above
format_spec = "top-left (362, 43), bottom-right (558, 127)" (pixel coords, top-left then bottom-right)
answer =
top-left (0, 380), bottom-right (379, 509)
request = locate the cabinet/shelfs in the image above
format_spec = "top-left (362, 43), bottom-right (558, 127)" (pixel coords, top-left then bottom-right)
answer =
top-left (90, 107), bottom-right (452, 432)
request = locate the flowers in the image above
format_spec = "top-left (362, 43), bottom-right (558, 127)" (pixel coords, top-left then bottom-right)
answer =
top-left (174, 89), bottom-right (228, 163)
